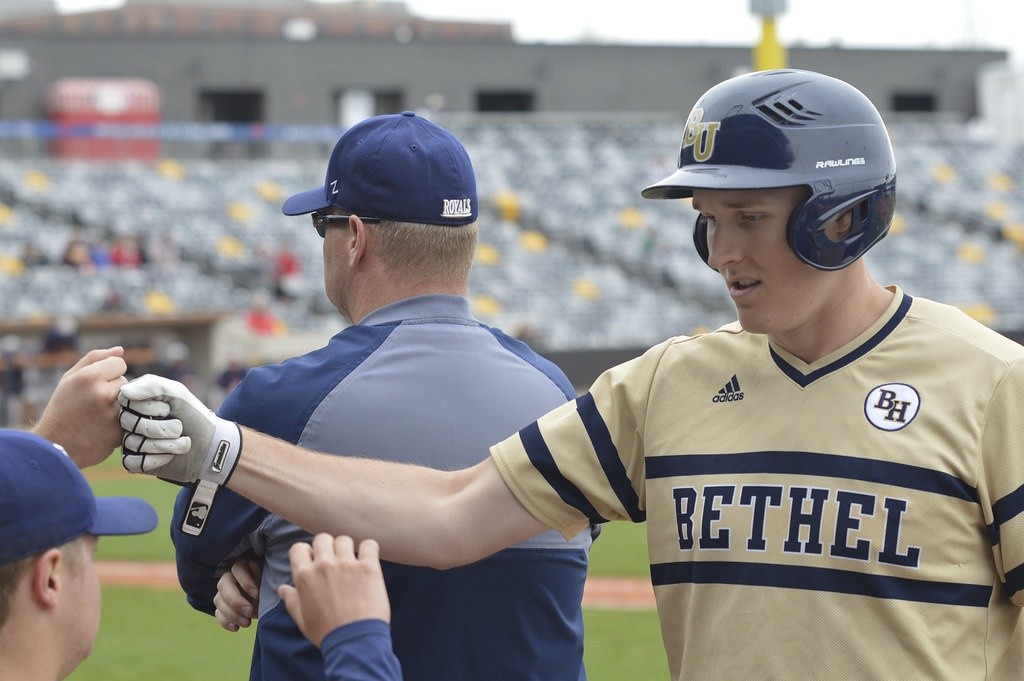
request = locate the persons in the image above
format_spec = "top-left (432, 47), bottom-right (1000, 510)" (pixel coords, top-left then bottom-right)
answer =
top-left (0, 345), bottom-right (404, 681)
top-left (118, 70), bottom-right (1024, 681)
top-left (169, 112), bottom-right (600, 681)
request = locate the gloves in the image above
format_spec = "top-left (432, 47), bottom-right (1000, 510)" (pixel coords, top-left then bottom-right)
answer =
top-left (118, 374), bottom-right (243, 538)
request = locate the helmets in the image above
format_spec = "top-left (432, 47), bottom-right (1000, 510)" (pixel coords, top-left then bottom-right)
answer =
top-left (641, 69), bottom-right (897, 272)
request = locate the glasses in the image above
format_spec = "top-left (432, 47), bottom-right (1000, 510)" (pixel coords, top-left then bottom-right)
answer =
top-left (311, 211), bottom-right (381, 239)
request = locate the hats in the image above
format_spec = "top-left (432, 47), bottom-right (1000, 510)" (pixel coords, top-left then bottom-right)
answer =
top-left (0, 427), bottom-right (158, 564)
top-left (282, 111), bottom-right (478, 227)
